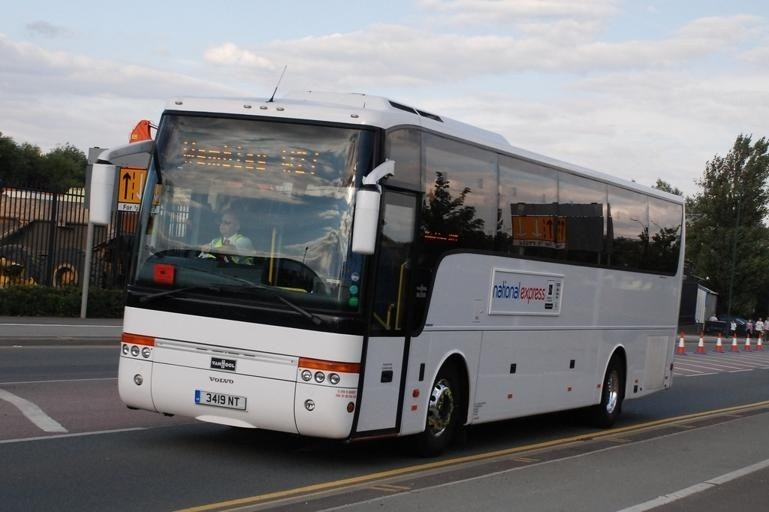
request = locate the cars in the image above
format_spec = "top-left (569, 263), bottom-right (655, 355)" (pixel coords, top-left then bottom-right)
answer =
top-left (717, 313), bottom-right (755, 334)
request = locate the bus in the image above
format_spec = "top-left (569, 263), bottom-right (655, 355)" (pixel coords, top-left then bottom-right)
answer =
top-left (99, 89), bottom-right (691, 456)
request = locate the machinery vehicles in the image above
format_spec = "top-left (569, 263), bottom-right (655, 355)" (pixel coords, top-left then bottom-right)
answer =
top-left (1, 188), bottom-right (140, 297)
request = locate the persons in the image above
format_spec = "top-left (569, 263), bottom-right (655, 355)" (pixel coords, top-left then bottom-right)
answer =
top-left (708, 312), bottom-right (720, 336)
top-left (762, 316), bottom-right (769, 342)
top-left (754, 317), bottom-right (764, 338)
top-left (730, 319), bottom-right (738, 338)
top-left (198, 209), bottom-right (256, 268)
top-left (745, 319), bottom-right (754, 339)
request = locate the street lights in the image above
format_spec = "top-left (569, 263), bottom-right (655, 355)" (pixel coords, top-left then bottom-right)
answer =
top-left (650, 218), bottom-right (662, 230)
top-left (626, 214), bottom-right (645, 232)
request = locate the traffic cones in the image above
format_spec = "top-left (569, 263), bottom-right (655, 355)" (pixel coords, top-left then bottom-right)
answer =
top-left (742, 333), bottom-right (753, 352)
top-left (756, 334), bottom-right (765, 352)
top-left (712, 332), bottom-right (725, 353)
top-left (728, 331), bottom-right (741, 353)
top-left (676, 331), bottom-right (686, 355)
top-left (695, 332), bottom-right (707, 354)
